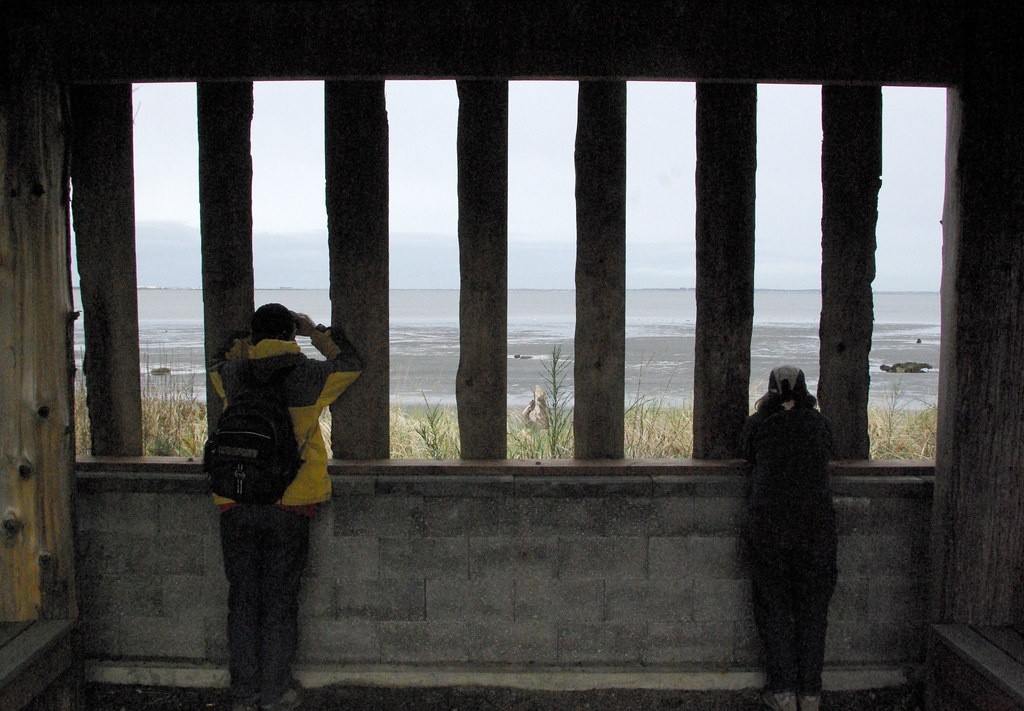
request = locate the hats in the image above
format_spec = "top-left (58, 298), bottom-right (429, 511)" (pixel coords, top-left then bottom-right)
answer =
top-left (769, 365), bottom-right (804, 393)
top-left (251, 304), bottom-right (294, 333)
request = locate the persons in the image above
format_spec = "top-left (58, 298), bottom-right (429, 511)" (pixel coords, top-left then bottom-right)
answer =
top-left (737, 363), bottom-right (838, 711)
top-left (208, 304), bottom-right (363, 711)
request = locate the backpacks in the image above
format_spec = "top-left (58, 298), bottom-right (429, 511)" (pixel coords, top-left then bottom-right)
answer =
top-left (203, 354), bottom-right (302, 505)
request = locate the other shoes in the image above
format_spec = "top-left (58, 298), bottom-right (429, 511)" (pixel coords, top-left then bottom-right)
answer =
top-left (229, 698), bottom-right (259, 711)
top-left (798, 694), bottom-right (821, 711)
top-left (259, 678), bottom-right (304, 711)
top-left (762, 683), bottom-right (797, 711)
